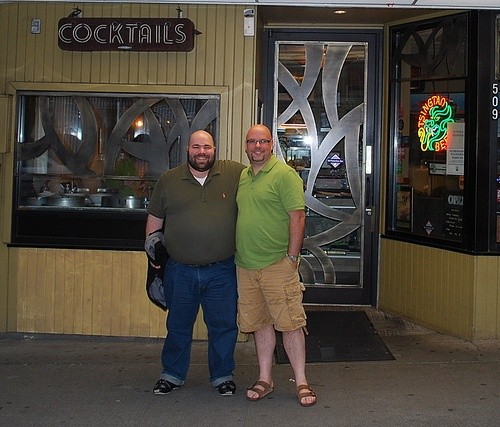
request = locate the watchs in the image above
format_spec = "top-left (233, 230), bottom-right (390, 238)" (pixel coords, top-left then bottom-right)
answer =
top-left (290, 256), bottom-right (297, 262)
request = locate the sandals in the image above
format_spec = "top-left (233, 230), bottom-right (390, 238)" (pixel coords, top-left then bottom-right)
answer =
top-left (296, 380), bottom-right (318, 406)
top-left (245, 379), bottom-right (275, 400)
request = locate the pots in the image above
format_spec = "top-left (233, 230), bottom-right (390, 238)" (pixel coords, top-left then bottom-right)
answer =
top-left (26, 193), bottom-right (85, 207)
top-left (119, 195), bottom-right (148, 210)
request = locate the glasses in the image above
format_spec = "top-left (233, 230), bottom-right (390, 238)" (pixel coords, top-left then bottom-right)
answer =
top-left (246, 138), bottom-right (273, 145)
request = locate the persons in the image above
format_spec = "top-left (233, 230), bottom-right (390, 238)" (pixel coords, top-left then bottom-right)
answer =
top-left (144, 130), bottom-right (248, 396)
top-left (236, 124), bottom-right (317, 407)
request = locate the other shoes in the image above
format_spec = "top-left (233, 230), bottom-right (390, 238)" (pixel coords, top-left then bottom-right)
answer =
top-left (152, 375), bottom-right (183, 395)
top-left (215, 378), bottom-right (236, 396)
